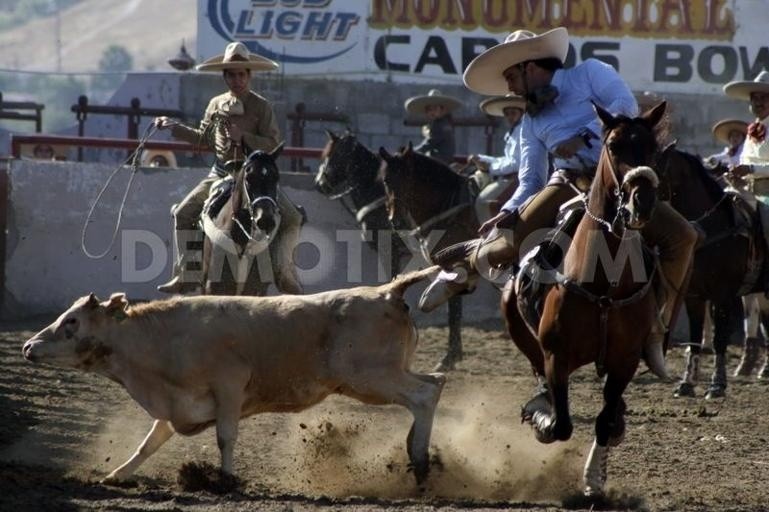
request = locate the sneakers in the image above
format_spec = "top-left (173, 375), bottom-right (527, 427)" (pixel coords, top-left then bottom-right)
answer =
top-left (419, 261), bottom-right (478, 312)
top-left (641, 342), bottom-right (666, 378)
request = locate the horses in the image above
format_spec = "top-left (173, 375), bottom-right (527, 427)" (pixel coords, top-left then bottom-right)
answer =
top-left (314, 126), bottom-right (405, 280)
top-left (658, 138), bottom-right (769, 403)
top-left (499, 96), bottom-right (673, 506)
top-left (201, 139), bottom-right (287, 297)
top-left (376, 140), bottom-right (519, 372)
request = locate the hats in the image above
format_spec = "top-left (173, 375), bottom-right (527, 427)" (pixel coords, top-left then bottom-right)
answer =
top-left (464, 26), bottom-right (570, 117)
top-left (713, 119), bottom-right (748, 144)
top-left (196, 42), bottom-right (279, 71)
top-left (404, 89), bottom-right (461, 115)
top-left (723, 71), bottom-right (769, 100)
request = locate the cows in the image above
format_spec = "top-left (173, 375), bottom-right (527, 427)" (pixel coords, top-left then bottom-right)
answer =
top-left (21, 241), bottom-right (477, 492)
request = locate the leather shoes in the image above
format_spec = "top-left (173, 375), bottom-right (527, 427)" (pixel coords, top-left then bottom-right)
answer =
top-left (283, 271), bottom-right (302, 294)
top-left (157, 275), bottom-right (198, 293)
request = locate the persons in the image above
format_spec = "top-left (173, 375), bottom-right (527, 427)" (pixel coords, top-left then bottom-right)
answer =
top-left (467, 107), bottom-right (522, 226)
top-left (150, 155), bottom-right (167, 167)
top-left (705, 129), bottom-right (745, 175)
top-left (730, 91), bottom-right (768, 244)
top-left (33, 143), bottom-right (54, 160)
top-left (154, 66), bottom-right (308, 294)
top-left (408, 102), bottom-right (455, 167)
top-left (471, 55), bottom-right (692, 420)
